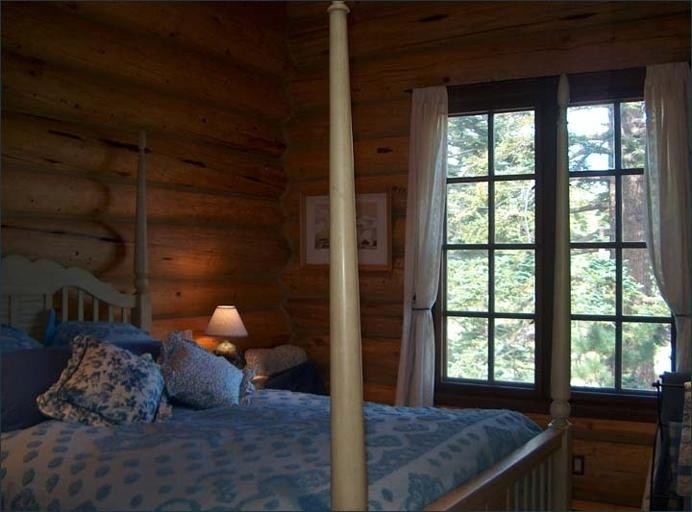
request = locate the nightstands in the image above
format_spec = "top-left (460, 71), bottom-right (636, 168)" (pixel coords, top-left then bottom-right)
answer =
top-left (250, 376), bottom-right (268, 390)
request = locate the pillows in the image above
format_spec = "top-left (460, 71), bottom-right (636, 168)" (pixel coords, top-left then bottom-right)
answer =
top-left (160, 330), bottom-right (255, 410)
top-left (51, 322), bottom-right (163, 373)
top-left (0, 324), bottom-right (61, 433)
top-left (35, 334), bottom-right (172, 428)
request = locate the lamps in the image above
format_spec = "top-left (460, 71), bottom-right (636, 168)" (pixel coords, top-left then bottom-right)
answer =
top-left (204, 305), bottom-right (248, 369)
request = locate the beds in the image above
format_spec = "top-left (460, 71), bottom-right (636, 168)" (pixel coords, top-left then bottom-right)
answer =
top-left (0, 256), bottom-right (574, 512)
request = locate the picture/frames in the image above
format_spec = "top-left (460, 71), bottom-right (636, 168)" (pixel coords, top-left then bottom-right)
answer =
top-left (299, 187), bottom-right (392, 273)
